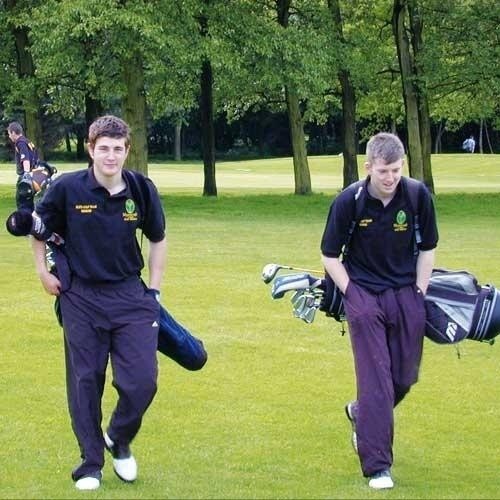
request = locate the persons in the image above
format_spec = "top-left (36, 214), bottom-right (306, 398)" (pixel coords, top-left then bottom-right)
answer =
top-left (7, 121), bottom-right (38, 212)
top-left (32, 115), bottom-right (167, 491)
top-left (318, 131), bottom-right (439, 489)
top-left (463, 135), bottom-right (476, 153)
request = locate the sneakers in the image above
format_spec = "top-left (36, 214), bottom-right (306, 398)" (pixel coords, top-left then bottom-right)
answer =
top-left (76, 469), bottom-right (103, 490)
top-left (368, 469), bottom-right (395, 489)
top-left (103, 432), bottom-right (138, 482)
top-left (345, 405), bottom-right (359, 456)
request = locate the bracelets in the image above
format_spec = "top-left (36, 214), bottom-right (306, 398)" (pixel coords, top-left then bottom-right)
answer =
top-left (149, 288), bottom-right (161, 304)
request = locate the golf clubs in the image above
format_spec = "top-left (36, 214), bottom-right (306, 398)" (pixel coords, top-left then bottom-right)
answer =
top-left (262, 263), bottom-right (325, 323)
